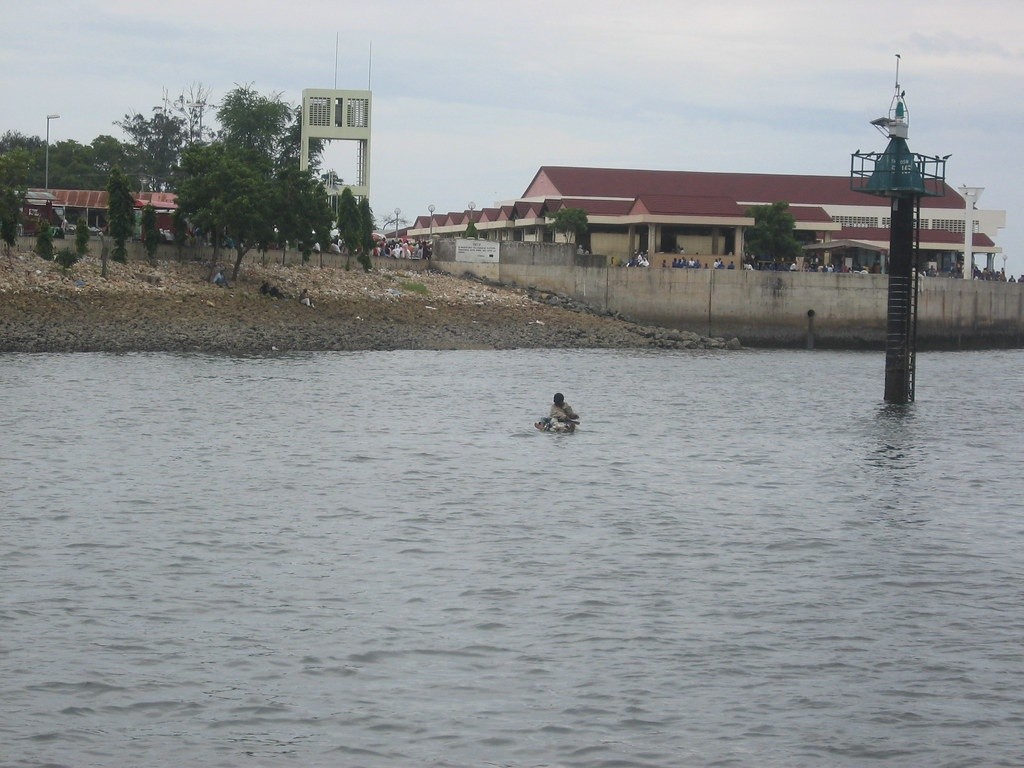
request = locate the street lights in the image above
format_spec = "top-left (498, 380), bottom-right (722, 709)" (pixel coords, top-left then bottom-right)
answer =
top-left (45, 114), bottom-right (61, 189)
top-left (468, 202), bottom-right (477, 220)
top-left (394, 207), bottom-right (402, 241)
top-left (1003, 253), bottom-right (1008, 273)
top-left (427, 204), bottom-right (436, 239)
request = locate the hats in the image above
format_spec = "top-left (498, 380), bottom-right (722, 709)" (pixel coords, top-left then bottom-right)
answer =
top-left (553, 393), bottom-right (564, 402)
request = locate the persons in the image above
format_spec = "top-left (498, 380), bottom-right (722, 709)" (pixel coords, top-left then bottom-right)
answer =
top-left (608, 249), bottom-right (650, 268)
top-left (714, 258), bottom-right (724, 269)
top-left (789, 261), bottom-right (797, 271)
top-left (671, 257), bottom-right (688, 268)
top-left (688, 258), bottom-right (695, 268)
top-left (585, 248), bottom-right (590, 254)
top-left (704, 264), bottom-right (709, 269)
top-left (159, 227), bottom-right (432, 260)
top-left (727, 261), bottom-right (735, 269)
top-left (745, 262), bottom-right (753, 270)
top-left (260, 280), bottom-right (316, 308)
top-left (671, 244), bottom-right (685, 254)
top-left (804, 262), bottom-right (870, 273)
top-left (694, 259), bottom-right (701, 268)
top-left (917, 261), bottom-right (1024, 282)
top-left (214, 269), bottom-right (233, 288)
top-left (661, 259), bottom-right (666, 267)
top-left (534, 393), bottom-right (579, 433)
top-left (576, 245), bottom-right (583, 254)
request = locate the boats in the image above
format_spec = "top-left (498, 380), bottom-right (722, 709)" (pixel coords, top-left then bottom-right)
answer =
top-left (534, 416), bottom-right (581, 433)
